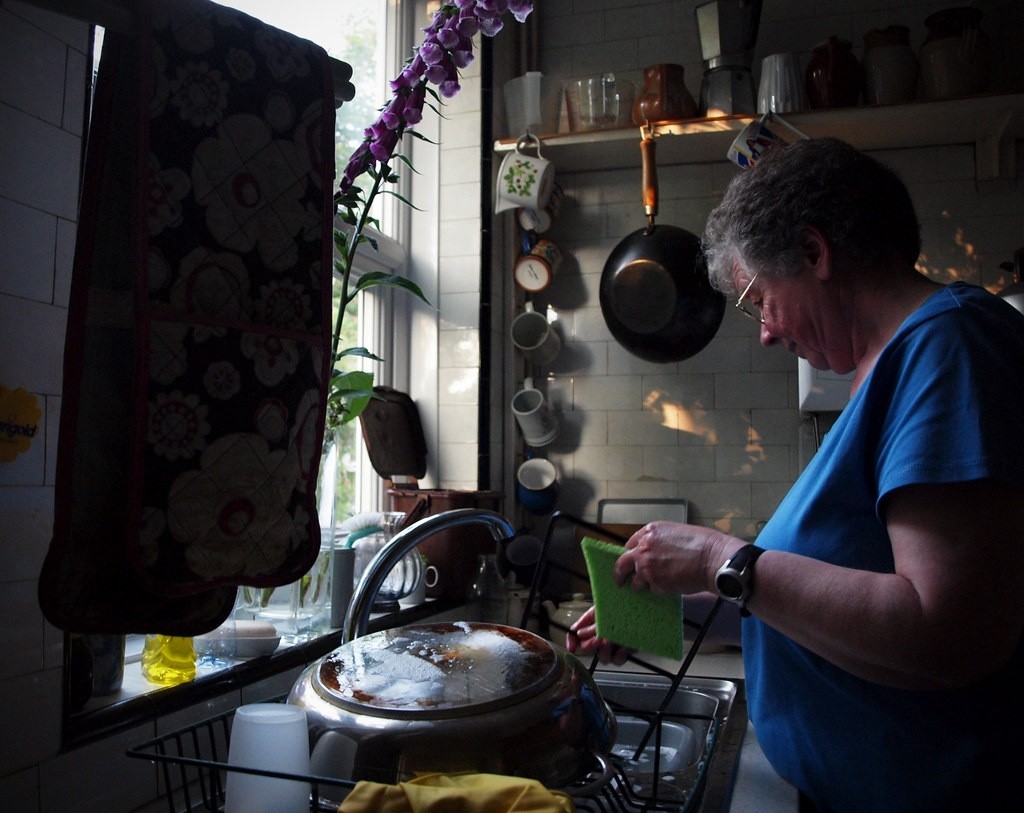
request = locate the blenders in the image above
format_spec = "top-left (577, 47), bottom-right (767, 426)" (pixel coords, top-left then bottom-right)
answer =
top-left (695, 0), bottom-right (763, 117)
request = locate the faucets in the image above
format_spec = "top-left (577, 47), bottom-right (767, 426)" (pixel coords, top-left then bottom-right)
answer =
top-left (339, 506), bottom-right (518, 647)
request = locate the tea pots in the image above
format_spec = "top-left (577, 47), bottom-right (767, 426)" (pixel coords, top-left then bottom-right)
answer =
top-left (542, 592), bottom-right (599, 656)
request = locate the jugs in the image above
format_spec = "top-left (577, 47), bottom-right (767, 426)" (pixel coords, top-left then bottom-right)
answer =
top-left (494, 133), bottom-right (555, 215)
top-left (342, 512), bottom-right (424, 611)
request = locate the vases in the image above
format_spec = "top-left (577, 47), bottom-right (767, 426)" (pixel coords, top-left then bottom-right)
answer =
top-left (238, 425), bottom-right (330, 620)
top-left (757, 0), bottom-right (989, 114)
top-left (631, 63), bottom-right (698, 125)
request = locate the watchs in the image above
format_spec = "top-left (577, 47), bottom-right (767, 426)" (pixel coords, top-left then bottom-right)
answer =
top-left (714, 544), bottom-right (766, 615)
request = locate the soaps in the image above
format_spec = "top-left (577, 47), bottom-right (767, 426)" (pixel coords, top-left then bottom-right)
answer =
top-left (192, 619), bottom-right (277, 640)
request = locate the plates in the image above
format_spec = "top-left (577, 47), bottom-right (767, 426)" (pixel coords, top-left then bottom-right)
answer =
top-left (197, 637), bottom-right (282, 657)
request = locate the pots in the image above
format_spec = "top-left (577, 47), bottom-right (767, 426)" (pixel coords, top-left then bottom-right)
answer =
top-left (599, 121), bottom-right (725, 363)
top-left (283, 620), bottom-right (618, 807)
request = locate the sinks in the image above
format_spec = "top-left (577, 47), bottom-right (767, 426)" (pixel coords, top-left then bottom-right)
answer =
top-left (589, 668), bottom-right (750, 782)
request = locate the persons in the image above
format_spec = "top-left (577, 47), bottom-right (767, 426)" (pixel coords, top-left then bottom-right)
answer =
top-left (567, 137), bottom-right (1024, 813)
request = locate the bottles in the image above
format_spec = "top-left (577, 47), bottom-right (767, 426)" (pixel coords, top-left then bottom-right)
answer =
top-left (468, 554), bottom-right (508, 625)
top-left (141, 632), bottom-right (196, 683)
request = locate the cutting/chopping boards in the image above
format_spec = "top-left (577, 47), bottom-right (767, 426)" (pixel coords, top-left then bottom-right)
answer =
top-left (571, 524), bottom-right (650, 599)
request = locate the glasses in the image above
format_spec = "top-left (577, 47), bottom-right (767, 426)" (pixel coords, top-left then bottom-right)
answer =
top-left (736, 262), bottom-right (767, 324)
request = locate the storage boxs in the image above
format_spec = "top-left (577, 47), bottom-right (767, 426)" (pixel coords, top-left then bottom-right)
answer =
top-left (355, 384), bottom-right (507, 598)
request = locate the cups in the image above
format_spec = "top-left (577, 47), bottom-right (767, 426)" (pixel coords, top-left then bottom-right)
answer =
top-left (566, 73), bottom-right (635, 133)
top-left (314, 545), bottom-right (356, 628)
top-left (513, 181), bottom-right (565, 291)
top-left (501, 68), bottom-right (563, 137)
top-left (510, 300), bottom-right (561, 367)
top-left (516, 448), bottom-right (559, 516)
top-left (727, 110), bottom-right (811, 171)
top-left (225, 703), bottom-right (312, 813)
top-left (758, 5), bottom-right (986, 114)
top-left (505, 534), bottom-right (540, 583)
top-left (631, 62), bottom-right (698, 126)
top-left (511, 376), bottom-right (560, 448)
top-left (503, 572), bottom-right (542, 634)
top-left (311, 732), bottom-right (356, 803)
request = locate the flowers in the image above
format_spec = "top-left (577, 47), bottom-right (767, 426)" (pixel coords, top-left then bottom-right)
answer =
top-left (238, 0), bottom-right (533, 606)
